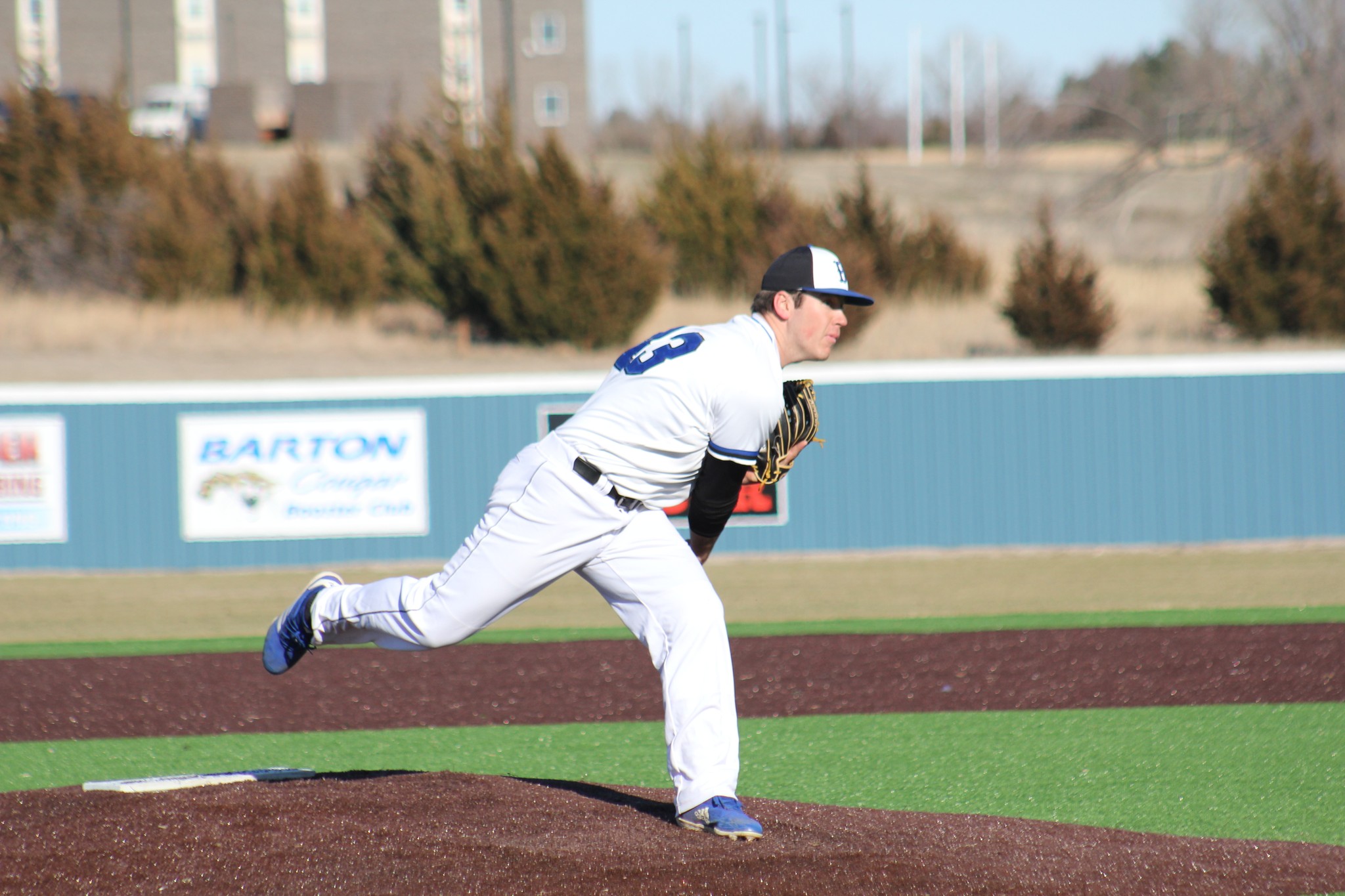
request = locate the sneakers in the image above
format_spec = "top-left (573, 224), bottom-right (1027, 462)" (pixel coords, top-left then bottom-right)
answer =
top-left (262, 571), bottom-right (344, 675)
top-left (676, 796), bottom-right (763, 841)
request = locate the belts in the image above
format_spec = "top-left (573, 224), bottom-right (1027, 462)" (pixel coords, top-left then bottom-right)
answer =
top-left (573, 457), bottom-right (642, 510)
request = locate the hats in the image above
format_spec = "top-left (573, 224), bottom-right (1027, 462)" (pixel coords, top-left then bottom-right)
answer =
top-left (761, 245), bottom-right (874, 306)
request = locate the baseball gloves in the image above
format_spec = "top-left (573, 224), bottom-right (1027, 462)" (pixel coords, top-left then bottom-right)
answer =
top-left (751, 380), bottom-right (818, 484)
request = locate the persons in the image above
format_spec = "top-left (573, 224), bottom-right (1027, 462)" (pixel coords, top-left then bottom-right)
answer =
top-left (260, 246), bottom-right (875, 842)
